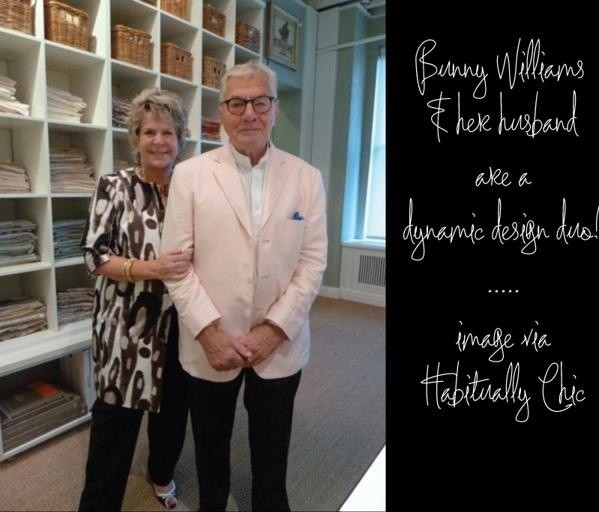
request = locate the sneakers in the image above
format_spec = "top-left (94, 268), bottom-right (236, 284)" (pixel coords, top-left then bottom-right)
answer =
top-left (153, 481), bottom-right (178, 509)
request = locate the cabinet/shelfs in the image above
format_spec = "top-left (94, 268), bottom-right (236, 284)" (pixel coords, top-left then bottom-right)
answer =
top-left (0, 1), bottom-right (266, 464)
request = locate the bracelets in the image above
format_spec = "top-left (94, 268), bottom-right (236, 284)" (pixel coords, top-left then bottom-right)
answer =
top-left (121, 257), bottom-right (137, 285)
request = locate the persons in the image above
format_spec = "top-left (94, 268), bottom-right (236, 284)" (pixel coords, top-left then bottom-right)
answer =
top-left (158, 58), bottom-right (328, 509)
top-left (77, 87), bottom-right (192, 510)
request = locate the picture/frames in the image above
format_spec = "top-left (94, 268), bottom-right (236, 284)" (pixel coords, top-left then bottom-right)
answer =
top-left (266, 1), bottom-right (301, 72)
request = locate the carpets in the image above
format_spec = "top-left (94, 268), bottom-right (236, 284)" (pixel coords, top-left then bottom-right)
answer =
top-left (1, 296), bottom-right (385, 511)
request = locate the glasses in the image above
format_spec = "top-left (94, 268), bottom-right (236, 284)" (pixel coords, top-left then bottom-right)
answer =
top-left (218, 94), bottom-right (278, 116)
